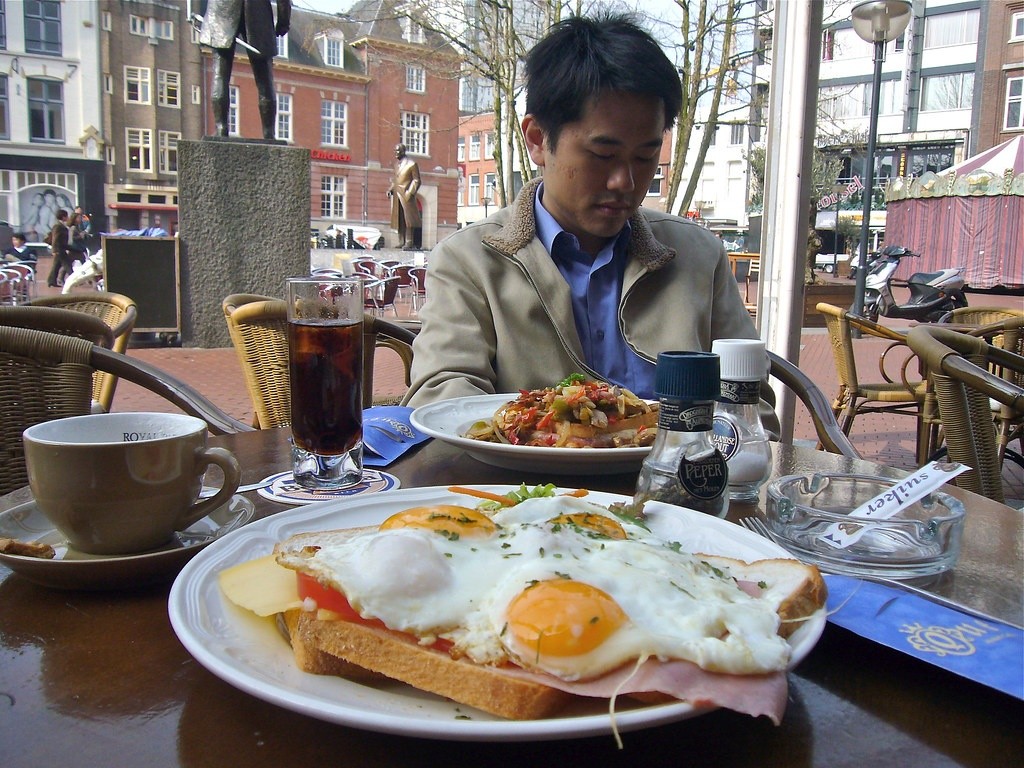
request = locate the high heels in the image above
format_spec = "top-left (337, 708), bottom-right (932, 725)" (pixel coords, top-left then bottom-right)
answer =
top-left (48, 283), bottom-right (62, 288)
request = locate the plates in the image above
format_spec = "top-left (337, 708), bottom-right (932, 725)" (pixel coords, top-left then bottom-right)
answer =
top-left (1, 486), bottom-right (256, 587)
top-left (408, 393), bottom-right (652, 475)
top-left (168, 484), bottom-right (827, 740)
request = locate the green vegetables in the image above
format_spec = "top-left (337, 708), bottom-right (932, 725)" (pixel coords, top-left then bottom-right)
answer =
top-left (428, 483), bottom-right (767, 599)
top-left (556, 372), bottom-right (586, 387)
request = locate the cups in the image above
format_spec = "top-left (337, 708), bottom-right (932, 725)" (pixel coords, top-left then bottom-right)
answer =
top-left (22, 412), bottom-right (241, 554)
top-left (283, 278), bottom-right (365, 490)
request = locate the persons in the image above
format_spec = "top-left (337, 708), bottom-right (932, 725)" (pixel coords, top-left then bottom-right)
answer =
top-left (47, 206), bottom-right (100, 286)
top-left (191, 0), bottom-right (291, 144)
top-left (385, 143), bottom-right (422, 250)
top-left (3, 234), bottom-right (37, 302)
top-left (398, 9), bottom-right (784, 441)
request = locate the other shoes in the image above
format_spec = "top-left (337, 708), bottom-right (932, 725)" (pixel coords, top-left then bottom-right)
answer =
top-left (58, 278), bottom-right (65, 285)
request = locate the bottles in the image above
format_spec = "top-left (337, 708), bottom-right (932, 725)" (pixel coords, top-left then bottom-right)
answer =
top-left (637, 350), bottom-right (729, 519)
top-left (712, 337), bottom-right (772, 499)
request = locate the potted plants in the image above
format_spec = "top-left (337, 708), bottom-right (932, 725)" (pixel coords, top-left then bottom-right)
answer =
top-left (741, 140), bottom-right (856, 325)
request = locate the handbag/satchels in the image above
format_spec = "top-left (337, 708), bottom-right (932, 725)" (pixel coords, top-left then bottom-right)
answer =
top-left (44, 231), bottom-right (52, 245)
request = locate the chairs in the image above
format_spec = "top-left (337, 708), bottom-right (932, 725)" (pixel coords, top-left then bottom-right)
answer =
top-left (741, 259), bottom-right (758, 316)
top-left (764, 301), bottom-right (1024, 507)
top-left (0, 261), bottom-right (38, 306)
top-left (221, 255), bottom-right (426, 430)
top-left (0, 291), bottom-right (258, 496)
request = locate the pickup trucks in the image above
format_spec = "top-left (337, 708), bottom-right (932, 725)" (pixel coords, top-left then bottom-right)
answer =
top-left (814, 253), bottom-right (850, 273)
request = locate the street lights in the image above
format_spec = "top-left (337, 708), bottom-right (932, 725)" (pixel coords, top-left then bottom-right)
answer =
top-left (850, 0), bottom-right (919, 339)
top-left (480, 195), bottom-right (492, 219)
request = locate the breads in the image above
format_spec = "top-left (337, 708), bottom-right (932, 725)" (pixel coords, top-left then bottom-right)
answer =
top-left (690, 551), bottom-right (826, 639)
top-left (280, 608), bottom-right (578, 720)
top-left (551, 410), bottom-right (659, 438)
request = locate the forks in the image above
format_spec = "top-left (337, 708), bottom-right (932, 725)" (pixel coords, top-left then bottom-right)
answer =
top-left (737, 516), bottom-right (778, 546)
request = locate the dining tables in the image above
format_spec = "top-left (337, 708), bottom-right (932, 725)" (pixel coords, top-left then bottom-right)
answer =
top-left (0, 427), bottom-right (1024, 768)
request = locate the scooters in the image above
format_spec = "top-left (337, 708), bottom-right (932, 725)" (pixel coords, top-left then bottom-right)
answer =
top-left (846, 244), bottom-right (971, 334)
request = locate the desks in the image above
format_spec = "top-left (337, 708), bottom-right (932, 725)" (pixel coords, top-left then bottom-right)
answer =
top-left (726, 252), bottom-right (760, 283)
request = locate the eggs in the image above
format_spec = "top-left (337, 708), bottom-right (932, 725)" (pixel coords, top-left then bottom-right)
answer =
top-left (271, 496), bottom-right (791, 684)
